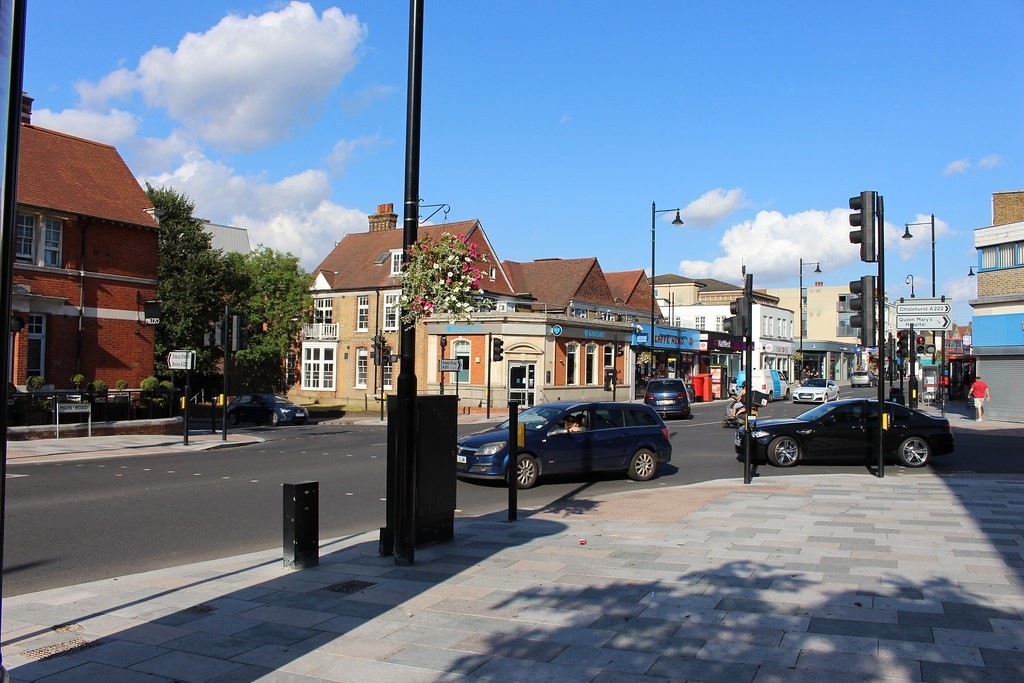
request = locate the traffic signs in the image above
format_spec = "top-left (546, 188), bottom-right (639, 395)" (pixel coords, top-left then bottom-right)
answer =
top-left (438, 358), bottom-right (464, 372)
top-left (166, 350), bottom-right (197, 370)
top-left (896, 302), bottom-right (953, 315)
top-left (896, 314), bottom-right (952, 332)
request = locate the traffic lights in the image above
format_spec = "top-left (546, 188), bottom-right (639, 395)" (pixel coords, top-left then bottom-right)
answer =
top-left (882, 413), bottom-right (890, 431)
top-left (884, 343), bottom-right (890, 357)
top-left (493, 338), bottom-right (504, 362)
top-left (369, 336), bottom-right (376, 358)
top-left (896, 330), bottom-right (910, 358)
top-left (849, 274), bottom-right (876, 347)
top-left (926, 344), bottom-right (936, 354)
top-left (380, 345), bottom-right (392, 366)
top-left (518, 422), bottom-right (527, 449)
top-left (916, 335), bottom-right (926, 354)
top-left (848, 190), bottom-right (877, 262)
top-left (612, 375), bottom-right (619, 386)
top-left (616, 340), bottom-right (623, 358)
top-left (722, 298), bottom-right (748, 337)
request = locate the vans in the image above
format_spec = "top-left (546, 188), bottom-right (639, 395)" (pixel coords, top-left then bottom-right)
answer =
top-left (737, 370), bottom-right (791, 402)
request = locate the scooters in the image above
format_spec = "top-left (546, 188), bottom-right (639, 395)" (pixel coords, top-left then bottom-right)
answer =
top-left (722, 389), bottom-right (758, 429)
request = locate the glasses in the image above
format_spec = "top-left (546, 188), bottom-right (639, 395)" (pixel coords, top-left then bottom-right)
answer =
top-left (567, 420), bottom-right (574, 423)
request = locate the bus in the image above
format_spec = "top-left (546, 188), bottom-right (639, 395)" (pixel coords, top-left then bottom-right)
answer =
top-left (946, 337), bottom-right (964, 357)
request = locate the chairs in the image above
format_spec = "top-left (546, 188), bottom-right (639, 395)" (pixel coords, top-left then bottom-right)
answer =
top-left (576, 415), bottom-right (587, 426)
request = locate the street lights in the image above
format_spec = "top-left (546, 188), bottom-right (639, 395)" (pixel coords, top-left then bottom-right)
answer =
top-left (901, 214), bottom-right (937, 366)
top-left (649, 200), bottom-right (684, 376)
top-left (797, 257), bottom-right (822, 382)
top-left (903, 274), bottom-right (916, 298)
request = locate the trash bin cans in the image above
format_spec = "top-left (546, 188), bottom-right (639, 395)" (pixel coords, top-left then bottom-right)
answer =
top-left (692, 376), bottom-right (704, 402)
top-left (685, 383), bottom-right (694, 403)
top-left (698, 374), bottom-right (713, 403)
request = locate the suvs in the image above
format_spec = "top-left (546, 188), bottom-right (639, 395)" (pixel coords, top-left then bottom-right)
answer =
top-left (644, 377), bottom-right (692, 419)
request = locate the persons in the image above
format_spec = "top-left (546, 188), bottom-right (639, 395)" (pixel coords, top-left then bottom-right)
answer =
top-left (564, 416), bottom-right (581, 432)
top-left (802, 366), bottom-right (819, 384)
top-left (968, 377), bottom-right (990, 421)
top-left (727, 382), bottom-right (747, 419)
top-left (857, 366), bottom-right (863, 370)
top-left (963, 371), bottom-right (970, 397)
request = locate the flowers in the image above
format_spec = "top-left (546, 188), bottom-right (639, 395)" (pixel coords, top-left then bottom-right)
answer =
top-left (392, 231), bottom-right (494, 332)
top-left (792, 352), bottom-right (802, 362)
top-left (635, 351), bottom-right (655, 367)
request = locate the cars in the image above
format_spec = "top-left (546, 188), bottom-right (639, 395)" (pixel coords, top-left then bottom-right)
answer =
top-left (736, 396), bottom-right (956, 469)
top-left (938, 365), bottom-right (949, 386)
top-left (792, 378), bottom-right (840, 404)
top-left (851, 370), bottom-right (878, 387)
top-left (227, 393), bottom-right (310, 427)
top-left (456, 400), bottom-right (673, 490)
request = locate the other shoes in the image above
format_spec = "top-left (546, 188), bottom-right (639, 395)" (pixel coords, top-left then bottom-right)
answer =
top-left (976, 419), bottom-right (982, 422)
